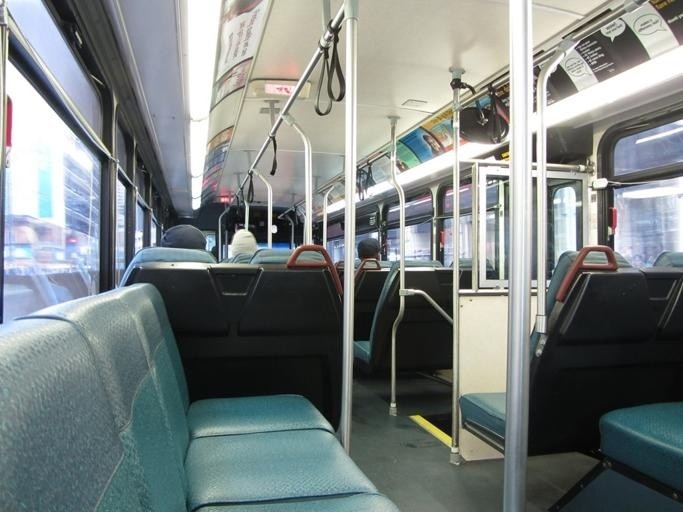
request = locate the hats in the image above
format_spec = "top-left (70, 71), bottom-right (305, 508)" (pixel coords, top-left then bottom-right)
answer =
top-left (357, 238), bottom-right (380, 258)
top-left (230, 229), bottom-right (256, 254)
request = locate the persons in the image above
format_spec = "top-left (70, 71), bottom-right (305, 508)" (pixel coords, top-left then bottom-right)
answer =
top-left (357, 238), bottom-right (381, 262)
top-left (423, 134), bottom-right (444, 157)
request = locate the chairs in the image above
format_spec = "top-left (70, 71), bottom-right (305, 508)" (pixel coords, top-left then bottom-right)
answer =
top-left (0, 246), bottom-right (681, 508)
